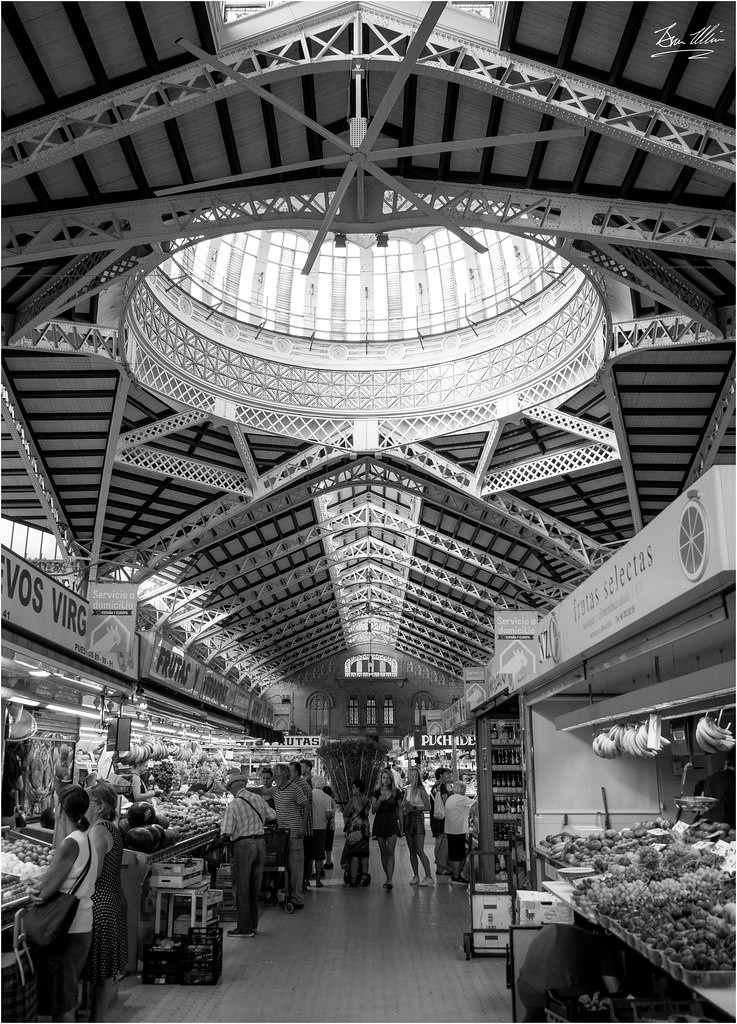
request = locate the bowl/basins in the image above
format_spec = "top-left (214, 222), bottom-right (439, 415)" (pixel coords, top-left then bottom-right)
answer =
top-left (556, 867), bottom-right (595, 884)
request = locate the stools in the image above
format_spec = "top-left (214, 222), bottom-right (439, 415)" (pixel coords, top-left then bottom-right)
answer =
top-left (155, 881), bottom-right (210, 939)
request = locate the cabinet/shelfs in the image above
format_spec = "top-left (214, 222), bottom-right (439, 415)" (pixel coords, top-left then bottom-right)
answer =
top-left (233, 747), bottom-right (331, 790)
top-left (418, 718), bottom-right (525, 881)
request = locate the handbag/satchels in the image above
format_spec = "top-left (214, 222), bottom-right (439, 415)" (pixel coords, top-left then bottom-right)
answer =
top-left (434, 792), bottom-right (447, 820)
top-left (21, 890), bottom-right (80, 951)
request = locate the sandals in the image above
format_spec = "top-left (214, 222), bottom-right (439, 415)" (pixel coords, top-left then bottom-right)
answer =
top-left (315, 882), bottom-right (325, 887)
top-left (437, 869), bottom-right (452, 875)
top-left (305, 882), bottom-right (311, 886)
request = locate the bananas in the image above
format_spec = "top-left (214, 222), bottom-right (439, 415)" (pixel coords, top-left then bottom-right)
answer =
top-left (592, 722), bottom-right (670, 759)
top-left (695, 717), bottom-right (736, 753)
top-left (122, 739), bottom-right (207, 764)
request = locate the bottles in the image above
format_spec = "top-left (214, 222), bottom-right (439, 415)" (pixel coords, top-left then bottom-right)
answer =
top-left (495, 823), bottom-right (516, 841)
top-left (493, 772), bottom-right (522, 787)
top-left (492, 749), bottom-right (521, 765)
top-left (495, 795), bottom-right (521, 814)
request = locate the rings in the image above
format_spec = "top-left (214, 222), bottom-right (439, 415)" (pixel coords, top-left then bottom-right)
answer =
top-left (30, 898), bottom-right (32, 901)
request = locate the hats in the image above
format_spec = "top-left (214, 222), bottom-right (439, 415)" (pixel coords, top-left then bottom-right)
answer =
top-left (223, 771), bottom-right (249, 789)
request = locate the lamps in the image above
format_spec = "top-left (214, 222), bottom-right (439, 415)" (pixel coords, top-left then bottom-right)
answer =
top-left (333, 232), bottom-right (347, 247)
top-left (375, 231), bottom-right (388, 247)
top-left (365, 566), bottom-right (374, 689)
top-left (110, 687), bottom-right (147, 711)
top-left (5, 703), bottom-right (37, 741)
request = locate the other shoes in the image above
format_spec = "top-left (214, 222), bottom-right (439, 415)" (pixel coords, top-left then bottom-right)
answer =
top-left (282, 902), bottom-right (310, 909)
top-left (227, 928), bottom-right (255, 937)
top-left (449, 877), bottom-right (471, 885)
top-left (419, 876), bottom-right (436, 886)
top-left (311, 872), bottom-right (326, 879)
top-left (324, 863), bottom-right (333, 870)
top-left (384, 882), bottom-right (393, 891)
top-left (410, 877), bottom-right (422, 884)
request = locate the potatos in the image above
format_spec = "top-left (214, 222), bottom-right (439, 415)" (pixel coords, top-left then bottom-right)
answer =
top-left (561, 818), bottom-right (673, 863)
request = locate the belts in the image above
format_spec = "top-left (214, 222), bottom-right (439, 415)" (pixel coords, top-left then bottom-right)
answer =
top-left (233, 834), bottom-right (265, 841)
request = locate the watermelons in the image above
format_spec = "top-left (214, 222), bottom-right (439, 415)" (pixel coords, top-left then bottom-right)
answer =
top-left (119, 802), bottom-right (179, 852)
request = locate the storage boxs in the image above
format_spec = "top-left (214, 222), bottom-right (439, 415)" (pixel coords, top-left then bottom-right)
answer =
top-left (466, 882), bottom-right (574, 956)
top-left (544, 987), bottom-right (695, 1023)
top-left (142, 856), bottom-right (238, 985)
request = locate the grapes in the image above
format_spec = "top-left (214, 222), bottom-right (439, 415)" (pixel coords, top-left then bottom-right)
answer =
top-left (572, 840), bottom-right (726, 915)
top-left (153, 761), bottom-right (222, 790)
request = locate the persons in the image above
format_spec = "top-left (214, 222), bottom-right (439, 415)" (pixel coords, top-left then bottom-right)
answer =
top-left (300, 758), bottom-right (313, 790)
top-left (262, 764), bottom-right (311, 910)
top-left (444, 781), bottom-right (477, 883)
top-left (403, 768), bottom-right (434, 887)
top-left (260, 768), bottom-right (278, 827)
top-left (81, 782), bottom-right (129, 1022)
top-left (307, 775), bottom-right (333, 888)
top-left (340, 779), bottom-right (371, 888)
top-left (323, 786), bottom-right (337, 870)
top-left (220, 772), bottom-right (276, 939)
top-left (1, 742), bottom-right (24, 829)
top-left (289, 762), bottom-right (313, 891)
top-left (372, 769), bottom-right (404, 891)
top-left (430, 768), bottom-right (453, 875)
top-left (130, 761), bottom-right (156, 806)
top-left (27, 784), bottom-right (98, 1023)
